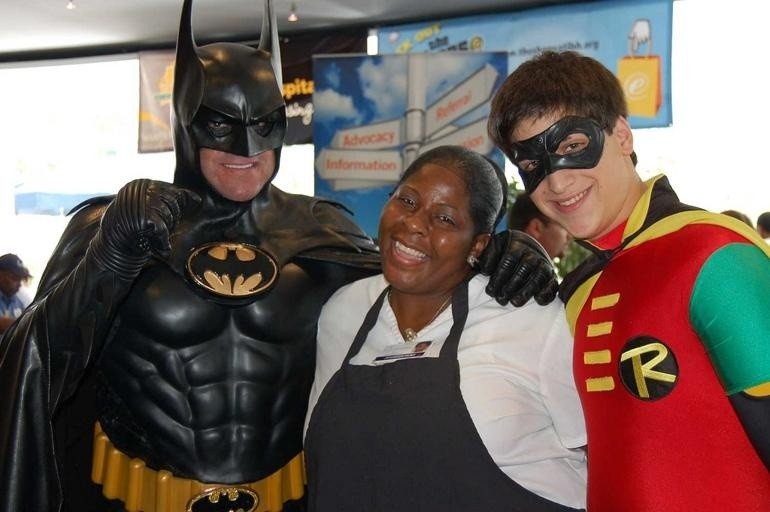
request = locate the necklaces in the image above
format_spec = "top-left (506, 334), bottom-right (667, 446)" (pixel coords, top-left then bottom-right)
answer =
top-left (386, 289), bottom-right (451, 341)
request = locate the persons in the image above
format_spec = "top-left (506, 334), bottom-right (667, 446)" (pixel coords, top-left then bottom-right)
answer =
top-left (301, 144), bottom-right (587, 512)
top-left (1, 41), bottom-right (560, 512)
top-left (509, 190), bottom-right (572, 262)
top-left (1, 252), bottom-right (34, 342)
top-left (486, 52), bottom-right (769, 511)
top-left (413, 342), bottom-right (431, 353)
top-left (756, 212), bottom-right (770, 245)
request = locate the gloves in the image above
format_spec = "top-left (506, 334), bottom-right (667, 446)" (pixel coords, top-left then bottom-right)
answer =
top-left (87, 179), bottom-right (201, 281)
top-left (479, 229), bottom-right (559, 308)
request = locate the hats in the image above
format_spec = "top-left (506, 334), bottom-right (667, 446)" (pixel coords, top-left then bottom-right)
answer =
top-left (0, 254), bottom-right (32, 278)
